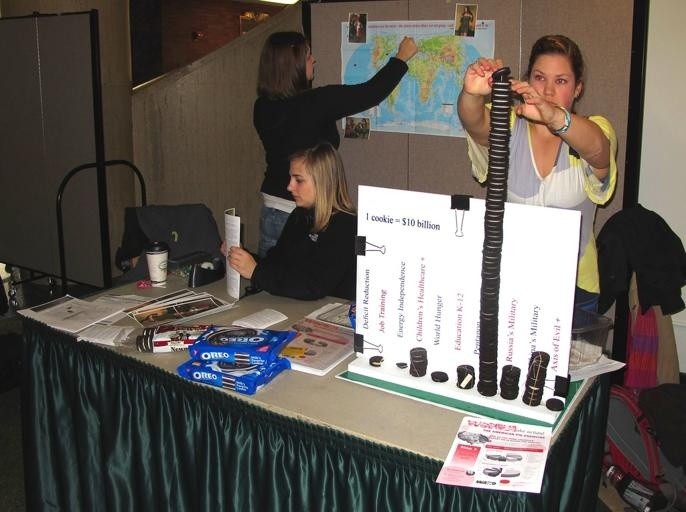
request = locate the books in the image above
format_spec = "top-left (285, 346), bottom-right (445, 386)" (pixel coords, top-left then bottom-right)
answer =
top-left (568, 306), bottom-right (611, 371)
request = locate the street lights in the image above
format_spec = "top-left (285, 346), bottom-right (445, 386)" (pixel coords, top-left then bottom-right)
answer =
top-left (117, 204), bottom-right (221, 260)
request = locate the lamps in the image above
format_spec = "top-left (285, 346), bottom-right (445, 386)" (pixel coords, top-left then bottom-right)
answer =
top-left (602, 205), bottom-right (686, 512)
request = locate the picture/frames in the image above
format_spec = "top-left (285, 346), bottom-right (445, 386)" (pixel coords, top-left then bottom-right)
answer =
top-left (19, 277), bottom-right (612, 512)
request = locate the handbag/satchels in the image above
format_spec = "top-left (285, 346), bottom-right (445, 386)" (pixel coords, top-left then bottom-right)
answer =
top-left (550, 105), bottom-right (571, 134)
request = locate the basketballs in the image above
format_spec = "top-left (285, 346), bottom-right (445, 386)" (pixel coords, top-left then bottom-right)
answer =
top-left (370, 355), bottom-right (385, 368)
top-left (455, 66), bottom-right (564, 411)
top-left (216, 352), bottom-right (257, 373)
top-left (396, 348), bottom-right (428, 377)
top-left (431, 371), bottom-right (449, 382)
top-left (136, 327), bottom-right (156, 353)
top-left (207, 326), bottom-right (258, 346)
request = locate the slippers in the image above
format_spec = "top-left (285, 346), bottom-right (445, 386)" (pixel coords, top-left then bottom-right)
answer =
top-left (603, 384), bottom-right (686, 512)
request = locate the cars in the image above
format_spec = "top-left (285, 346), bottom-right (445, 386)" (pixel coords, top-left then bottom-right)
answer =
top-left (144, 242), bottom-right (169, 289)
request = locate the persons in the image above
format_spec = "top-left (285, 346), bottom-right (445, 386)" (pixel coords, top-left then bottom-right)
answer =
top-left (346, 118), bottom-right (367, 138)
top-left (458, 35), bottom-right (618, 314)
top-left (460, 5), bottom-right (474, 30)
top-left (220, 141), bottom-right (356, 300)
top-left (253, 31), bottom-right (423, 261)
